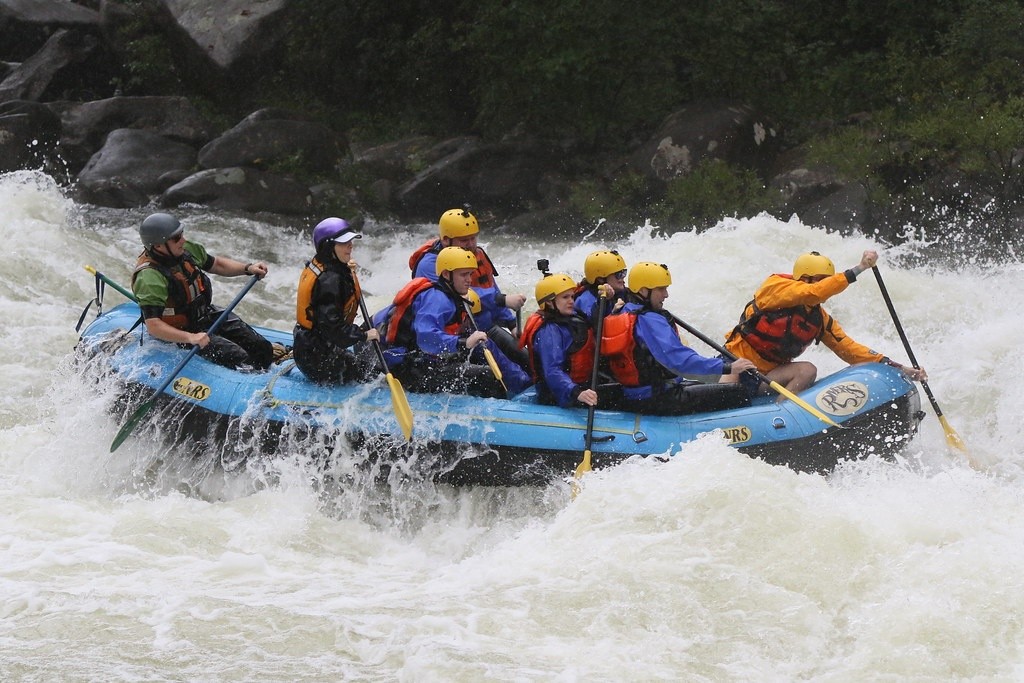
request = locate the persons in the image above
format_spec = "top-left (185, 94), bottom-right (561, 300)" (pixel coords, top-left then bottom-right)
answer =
top-left (573, 251), bottom-right (628, 327)
top-left (716, 249), bottom-right (928, 403)
top-left (292, 217), bottom-right (381, 385)
top-left (353, 208), bottom-right (532, 399)
top-left (518, 274), bottom-right (629, 411)
top-left (599, 261), bottom-right (756, 415)
top-left (131, 212), bottom-right (274, 372)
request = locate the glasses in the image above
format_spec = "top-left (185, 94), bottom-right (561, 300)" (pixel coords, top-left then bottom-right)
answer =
top-left (169, 231), bottom-right (183, 242)
top-left (612, 270), bottom-right (627, 279)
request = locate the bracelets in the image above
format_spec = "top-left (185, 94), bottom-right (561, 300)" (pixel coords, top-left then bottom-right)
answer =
top-left (245, 263), bottom-right (252, 275)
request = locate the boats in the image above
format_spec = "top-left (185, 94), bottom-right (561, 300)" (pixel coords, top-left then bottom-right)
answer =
top-left (74, 265), bottom-right (926, 488)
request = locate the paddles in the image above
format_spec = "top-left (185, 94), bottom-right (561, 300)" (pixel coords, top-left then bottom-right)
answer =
top-left (350, 262), bottom-right (415, 443)
top-left (573, 284), bottom-right (608, 500)
top-left (516, 308), bottom-right (522, 338)
top-left (669, 312), bottom-right (846, 430)
top-left (109, 274), bottom-right (259, 455)
top-left (83, 262), bottom-right (136, 304)
top-left (871, 261), bottom-right (965, 449)
top-left (466, 300), bottom-right (508, 393)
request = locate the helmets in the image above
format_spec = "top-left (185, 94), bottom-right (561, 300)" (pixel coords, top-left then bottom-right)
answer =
top-left (462, 288), bottom-right (481, 313)
top-left (313, 216), bottom-right (354, 251)
top-left (627, 261), bottom-right (671, 293)
top-left (584, 251), bottom-right (628, 284)
top-left (793, 253), bottom-right (835, 281)
top-left (536, 273), bottom-right (577, 310)
top-left (435, 246), bottom-right (479, 276)
top-left (139, 213), bottom-right (185, 251)
top-left (438, 209), bottom-right (479, 240)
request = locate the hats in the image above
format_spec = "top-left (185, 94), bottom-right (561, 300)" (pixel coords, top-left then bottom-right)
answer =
top-left (331, 230), bottom-right (362, 243)
